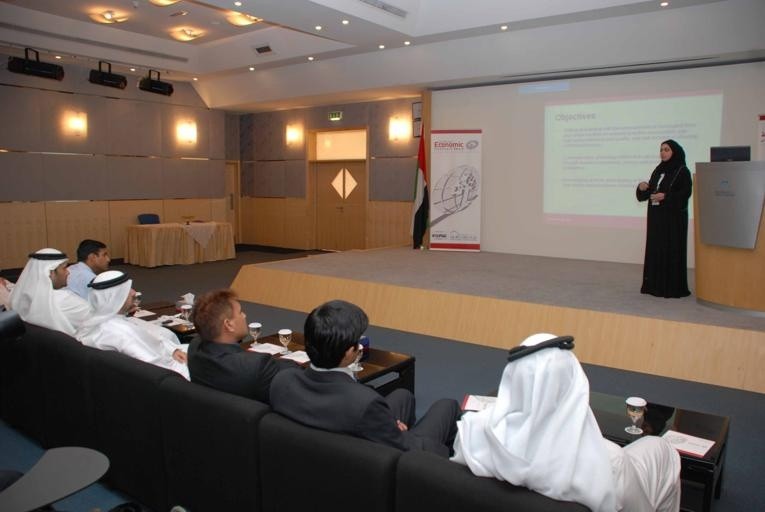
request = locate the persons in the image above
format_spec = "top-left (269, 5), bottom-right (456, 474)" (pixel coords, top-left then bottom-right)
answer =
top-left (60, 238), bottom-right (110, 301)
top-left (445, 331), bottom-right (683, 511)
top-left (70, 270), bottom-right (189, 377)
top-left (3, 247), bottom-right (92, 337)
top-left (636, 139), bottom-right (692, 299)
top-left (187, 287), bottom-right (302, 405)
top-left (268, 299), bottom-right (463, 459)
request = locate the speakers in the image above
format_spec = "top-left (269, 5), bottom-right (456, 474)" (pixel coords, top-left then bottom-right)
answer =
top-left (7, 56), bottom-right (173, 96)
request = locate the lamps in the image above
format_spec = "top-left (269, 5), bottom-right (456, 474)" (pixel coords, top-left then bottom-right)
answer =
top-left (169, 28), bottom-right (206, 41)
top-left (7, 48), bottom-right (63, 80)
top-left (89, 60), bottom-right (127, 89)
top-left (149, 0), bottom-right (180, 7)
top-left (87, 10), bottom-right (128, 25)
top-left (225, 12), bottom-right (263, 27)
top-left (139, 68), bottom-right (174, 96)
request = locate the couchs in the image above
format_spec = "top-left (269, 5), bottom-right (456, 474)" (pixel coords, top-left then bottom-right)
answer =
top-left (0, 303), bottom-right (594, 512)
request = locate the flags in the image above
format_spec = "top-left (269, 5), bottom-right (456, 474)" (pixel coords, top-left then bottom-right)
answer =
top-left (410, 123), bottom-right (430, 250)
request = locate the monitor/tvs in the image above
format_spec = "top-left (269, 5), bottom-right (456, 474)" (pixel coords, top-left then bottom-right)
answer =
top-left (710, 146), bottom-right (751, 161)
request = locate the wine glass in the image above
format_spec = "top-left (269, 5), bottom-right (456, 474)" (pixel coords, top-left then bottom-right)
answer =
top-left (248, 322), bottom-right (262, 347)
top-left (625, 396), bottom-right (647, 435)
top-left (180, 304), bottom-right (195, 330)
top-left (132, 292), bottom-right (143, 314)
top-left (279, 329), bottom-right (293, 354)
top-left (348, 342), bottom-right (364, 371)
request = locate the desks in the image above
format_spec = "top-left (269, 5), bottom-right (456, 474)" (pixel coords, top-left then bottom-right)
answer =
top-left (124, 221), bottom-right (236, 267)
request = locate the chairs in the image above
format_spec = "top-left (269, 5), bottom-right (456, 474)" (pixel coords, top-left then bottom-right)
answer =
top-left (138, 213), bottom-right (160, 224)
